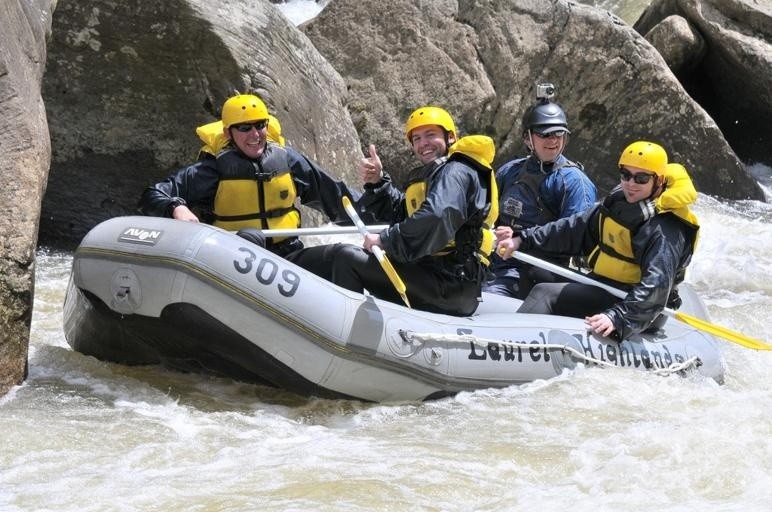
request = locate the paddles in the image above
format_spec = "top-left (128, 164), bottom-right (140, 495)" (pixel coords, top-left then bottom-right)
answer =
top-left (499, 247), bottom-right (772, 351)
top-left (342, 195), bottom-right (411, 308)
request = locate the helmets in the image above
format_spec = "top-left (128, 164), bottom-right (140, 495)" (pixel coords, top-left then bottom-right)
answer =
top-left (522, 102), bottom-right (572, 133)
top-left (221, 95), bottom-right (269, 127)
top-left (405, 105), bottom-right (461, 141)
top-left (618, 141), bottom-right (668, 176)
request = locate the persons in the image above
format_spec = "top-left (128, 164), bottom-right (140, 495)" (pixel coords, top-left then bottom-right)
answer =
top-left (496, 140), bottom-right (700, 343)
top-left (362, 105), bottom-right (500, 316)
top-left (135, 93), bottom-right (390, 294)
top-left (482, 102), bottom-right (598, 296)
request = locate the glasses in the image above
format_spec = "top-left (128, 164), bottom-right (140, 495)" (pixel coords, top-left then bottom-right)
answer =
top-left (534, 131), bottom-right (567, 138)
top-left (618, 168), bottom-right (656, 183)
top-left (229, 120), bottom-right (267, 131)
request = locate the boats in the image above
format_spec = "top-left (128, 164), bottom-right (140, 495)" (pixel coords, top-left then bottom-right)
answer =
top-left (63, 216), bottom-right (726, 406)
